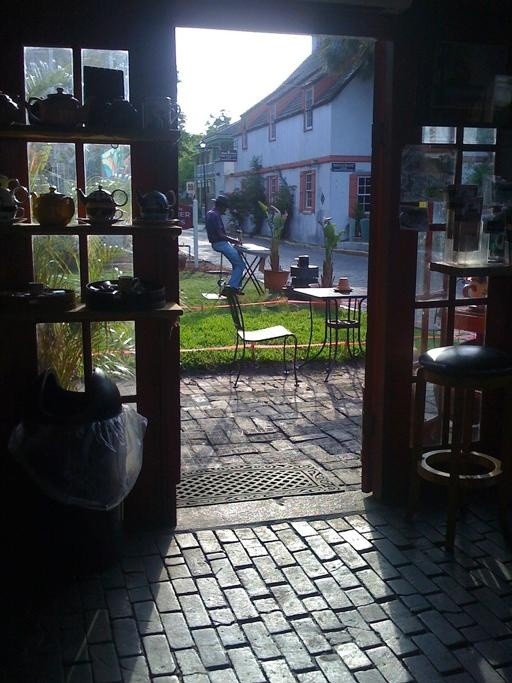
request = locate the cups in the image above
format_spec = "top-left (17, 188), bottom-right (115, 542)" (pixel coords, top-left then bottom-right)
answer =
top-left (27, 282), bottom-right (45, 294)
top-left (117, 275), bottom-right (139, 296)
top-left (336, 279), bottom-right (350, 290)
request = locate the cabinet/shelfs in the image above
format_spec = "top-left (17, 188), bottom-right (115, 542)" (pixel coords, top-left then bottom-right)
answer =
top-left (1, 2), bottom-right (180, 532)
top-left (382, 33), bottom-right (510, 508)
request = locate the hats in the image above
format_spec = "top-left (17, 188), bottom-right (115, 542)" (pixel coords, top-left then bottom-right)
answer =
top-left (210, 194), bottom-right (231, 208)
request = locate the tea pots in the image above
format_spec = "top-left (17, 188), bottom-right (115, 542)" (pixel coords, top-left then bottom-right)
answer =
top-left (132, 95), bottom-right (180, 127)
top-left (136, 188), bottom-right (176, 219)
top-left (26, 88), bottom-right (85, 133)
top-left (79, 185), bottom-right (128, 218)
top-left (31, 187), bottom-right (75, 223)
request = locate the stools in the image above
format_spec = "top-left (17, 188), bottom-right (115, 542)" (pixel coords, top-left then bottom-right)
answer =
top-left (208, 251), bottom-right (228, 297)
top-left (406, 347), bottom-right (511, 555)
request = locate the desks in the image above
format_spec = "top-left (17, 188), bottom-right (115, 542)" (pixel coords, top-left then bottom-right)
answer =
top-left (293, 286), bottom-right (369, 382)
top-left (234, 243), bottom-right (271, 296)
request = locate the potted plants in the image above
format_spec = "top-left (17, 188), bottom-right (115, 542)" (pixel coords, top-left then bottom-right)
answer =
top-left (351, 202), bottom-right (364, 241)
top-left (309, 218), bottom-right (346, 312)
top-left (258, 200), bottom-right (290, 291)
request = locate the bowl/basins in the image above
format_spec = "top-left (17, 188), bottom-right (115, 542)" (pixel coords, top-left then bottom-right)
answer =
top-left (37, 288), bottom-right (76, 311)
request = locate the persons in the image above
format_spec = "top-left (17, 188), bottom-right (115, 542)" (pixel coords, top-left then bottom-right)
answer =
top-left (204, 195), bottom-right (246, 297)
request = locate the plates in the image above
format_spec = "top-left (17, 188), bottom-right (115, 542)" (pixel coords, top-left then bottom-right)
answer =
top-left (334, 288), bottom-right (353, 292)
top-left (76, 216), bottom-right (125, 225)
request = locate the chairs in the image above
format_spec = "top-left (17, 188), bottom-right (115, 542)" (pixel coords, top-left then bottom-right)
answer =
top-left (414, 307), bottom-right (443, 347)
top-left (224, 286), bottom-right (299, 389)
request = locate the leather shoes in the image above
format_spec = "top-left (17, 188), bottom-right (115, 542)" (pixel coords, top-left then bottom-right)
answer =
top-left (230, 286), bottom-right (245, 295)
